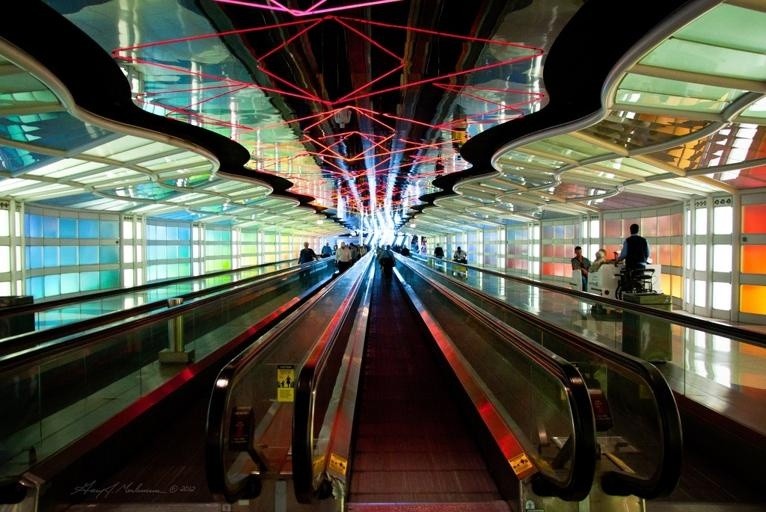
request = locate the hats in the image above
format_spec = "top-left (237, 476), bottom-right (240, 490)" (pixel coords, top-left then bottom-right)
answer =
top-left (341, 242), bottom-right (345, 247)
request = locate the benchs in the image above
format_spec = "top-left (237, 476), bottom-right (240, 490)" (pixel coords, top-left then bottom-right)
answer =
top-left (587, 263), bottom-right (662, 305)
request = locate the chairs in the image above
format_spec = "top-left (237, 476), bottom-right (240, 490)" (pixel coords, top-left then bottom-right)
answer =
top-left (571, 268), bottom-right (587, 291)
top-left (614, 269), bottom-right (655, 300)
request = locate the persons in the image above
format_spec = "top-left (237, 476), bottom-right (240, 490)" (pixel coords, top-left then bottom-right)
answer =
top-left (454, 245), bottom-right (468, 261)
top-left (411, 235), bottom-right (428, 257)
top-left (322, 241), bottom-right (412, 289)
top-left (434, 242), bottom-right (444, 270)
top-left (571, 245), bottom-right (592, 291)
top-left (298, 242), bottom-right (320, 289)
top-left (612, 224), bottom-right (649, 301)
top-left (588, 248), bottom-right (625, 274)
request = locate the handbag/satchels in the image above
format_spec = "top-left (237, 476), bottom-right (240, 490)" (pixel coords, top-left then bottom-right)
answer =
top-left (379, 256), bottom-right (396, 267)
